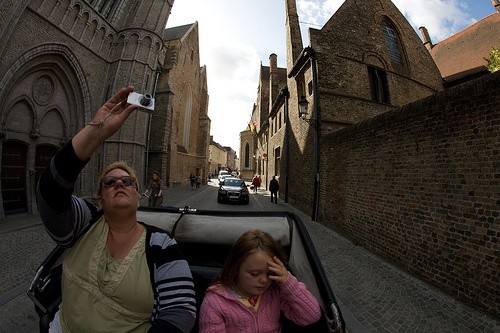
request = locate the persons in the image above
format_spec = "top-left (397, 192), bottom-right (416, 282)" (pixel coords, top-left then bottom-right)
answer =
top-left (143, 171), bottom-right (164, 207)
top-left (199, 229), bottom-right (321, 333)
top-left (188, 172), bottom-right (196, 190)
top-left (269, 175), bottom-right (279, 205)
top-left (251, 175), bottom-right (260, 194)
top-left (195, 173), bottom-right (202, 191)
top-left (39, 85), bottom-right (197, 333)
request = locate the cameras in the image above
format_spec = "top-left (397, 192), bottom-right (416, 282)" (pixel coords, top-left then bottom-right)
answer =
top-left (125, 91), bottom-right (156, 113)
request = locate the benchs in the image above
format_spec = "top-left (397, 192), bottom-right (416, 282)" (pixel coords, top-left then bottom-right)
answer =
top-left (39, 267), bottom-right (326, 333)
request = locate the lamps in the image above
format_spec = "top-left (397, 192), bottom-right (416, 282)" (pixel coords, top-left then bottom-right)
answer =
top-left (297, 96), bottom-right (320, 129)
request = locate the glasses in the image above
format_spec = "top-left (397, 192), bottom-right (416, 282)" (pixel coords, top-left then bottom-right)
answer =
top-left (99, 176), bottom-right (136, 189)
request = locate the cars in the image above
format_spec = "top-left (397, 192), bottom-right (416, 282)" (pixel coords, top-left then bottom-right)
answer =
top-left (217, 169), bottom-right (250, 204)
